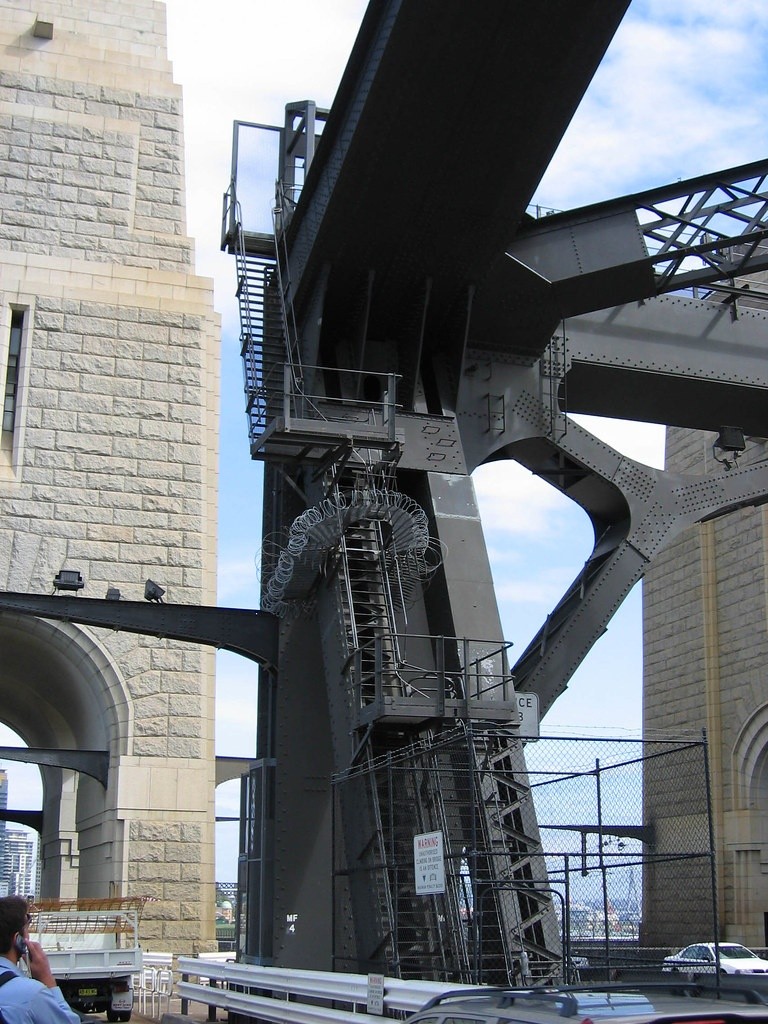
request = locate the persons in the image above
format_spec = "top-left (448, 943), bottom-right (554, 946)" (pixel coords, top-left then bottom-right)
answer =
top-left (0, 895), bottom-right (81, 1024)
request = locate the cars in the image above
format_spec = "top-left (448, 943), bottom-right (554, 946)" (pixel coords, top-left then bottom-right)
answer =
top-left (661, 942), bottom-right (768, 975)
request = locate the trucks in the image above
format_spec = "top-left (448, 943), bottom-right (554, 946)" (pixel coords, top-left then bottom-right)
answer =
top-left (15, 910), bottom-right (143, 1023)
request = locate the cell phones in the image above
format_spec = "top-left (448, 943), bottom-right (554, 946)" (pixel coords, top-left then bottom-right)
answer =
top-left (15, 935), bottom-right (27, 956)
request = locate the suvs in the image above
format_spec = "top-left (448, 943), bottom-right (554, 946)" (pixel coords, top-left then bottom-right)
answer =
top-left (402, 982), bottom-right (768, 1024)
